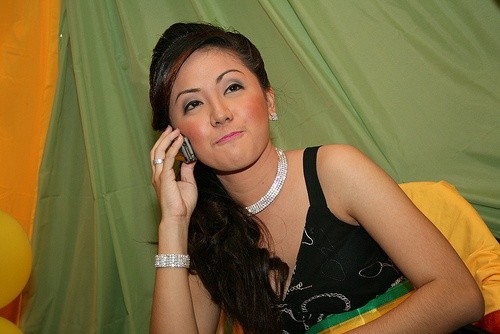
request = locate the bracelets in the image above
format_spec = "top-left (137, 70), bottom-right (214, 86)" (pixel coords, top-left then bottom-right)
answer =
top-left (154, 254), bottom-right (191, 268)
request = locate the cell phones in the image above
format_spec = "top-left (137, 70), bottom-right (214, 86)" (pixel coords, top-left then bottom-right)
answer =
top-left (160, 129), bottom-right (195, 164)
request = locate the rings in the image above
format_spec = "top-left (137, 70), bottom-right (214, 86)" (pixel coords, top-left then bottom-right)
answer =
top-left (153, 159), bottom-right (165, 164)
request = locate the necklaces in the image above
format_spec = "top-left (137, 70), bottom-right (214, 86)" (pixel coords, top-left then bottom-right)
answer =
top-left (245, 147), bottom-right (289, 215)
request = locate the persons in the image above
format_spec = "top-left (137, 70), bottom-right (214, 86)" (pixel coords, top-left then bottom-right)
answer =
top-left (149, 22), bottom-right (485, 334)
